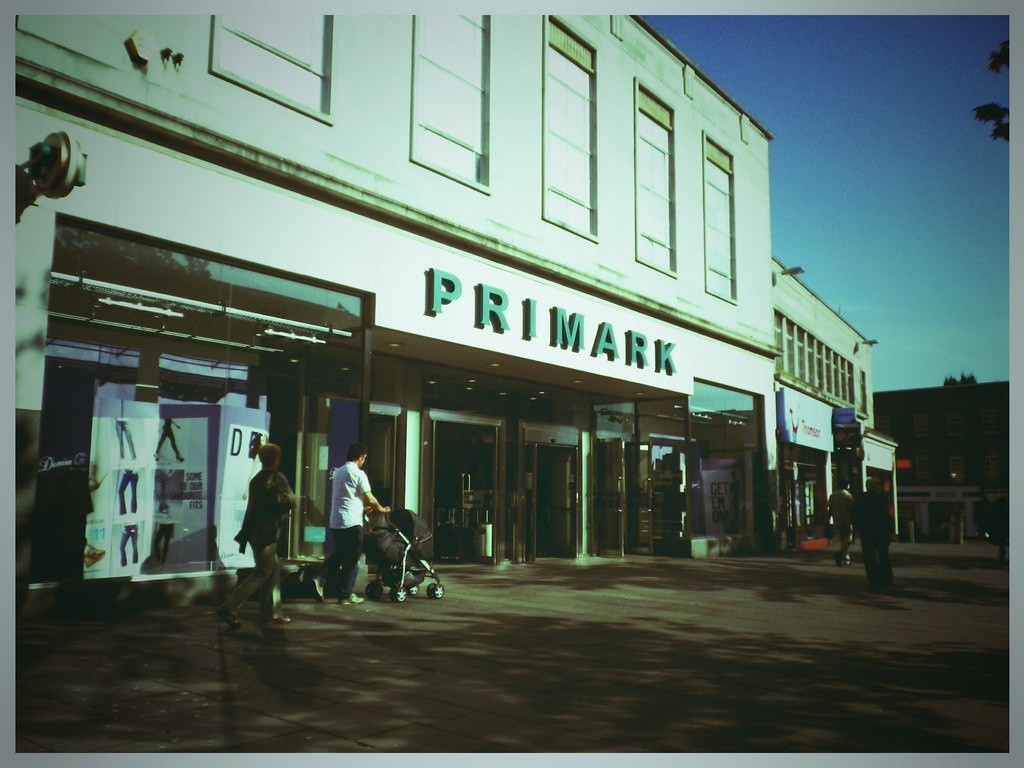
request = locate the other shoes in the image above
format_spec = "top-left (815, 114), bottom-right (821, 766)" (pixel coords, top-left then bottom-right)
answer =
top-left (834, 552), bottom-right (851, 567)
top-left (261, 615), bottom-right (291, 625)
top-left (216, 606), bottom-right (242, 629)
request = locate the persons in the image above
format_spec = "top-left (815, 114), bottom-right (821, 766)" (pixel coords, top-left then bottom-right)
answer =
top-left (215, 443), bottom-right (298, 628)
top-left (823, 479), bottom-right (893, 593)
top-left (323, 442), bottom-right (391, 606)
top-left (114, 416), bottom-right (184, 567)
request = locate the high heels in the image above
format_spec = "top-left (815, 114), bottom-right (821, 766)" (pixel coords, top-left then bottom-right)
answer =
top-left (153, 454), bottom-right (184, 462)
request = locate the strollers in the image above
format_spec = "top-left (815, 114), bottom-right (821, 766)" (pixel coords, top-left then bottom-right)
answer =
top-left (363, 507), bottom-right (445, 603)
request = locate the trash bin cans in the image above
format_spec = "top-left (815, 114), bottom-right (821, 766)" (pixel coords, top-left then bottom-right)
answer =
top-left (476, 521), bottom-right (493, 563)
top-left (949, 512), bottom-right (966, 544)
top-left (903, 521), bottom-right (915, 542)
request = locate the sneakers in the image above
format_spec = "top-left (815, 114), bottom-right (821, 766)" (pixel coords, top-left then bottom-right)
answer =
top-left (311, 577), bottom-right (325, 602)
top-left (340, 592), bottom-right (368, 607)
